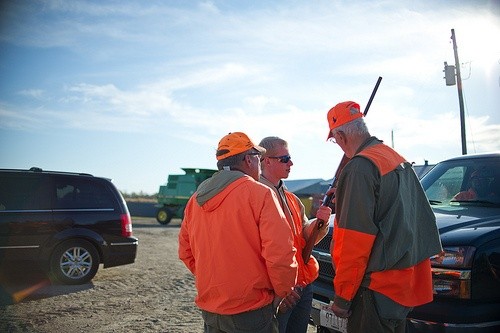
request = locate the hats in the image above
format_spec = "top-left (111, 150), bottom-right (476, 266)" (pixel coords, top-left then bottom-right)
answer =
top-left (326, 101), bottom-right (362, 142)
top-left (216, 132), bottom-right (261, 160)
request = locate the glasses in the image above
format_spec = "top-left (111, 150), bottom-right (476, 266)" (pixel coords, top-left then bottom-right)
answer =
top-left (243, 151), bottom-right (260, 161)
top-left (330, 131), bottom-right (343, 143)
top-left (269, 154), bottom-right (291, 163)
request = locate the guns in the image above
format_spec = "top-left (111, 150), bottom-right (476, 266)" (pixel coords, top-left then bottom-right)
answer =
top-left (272, 75), bottom-right (382, 333)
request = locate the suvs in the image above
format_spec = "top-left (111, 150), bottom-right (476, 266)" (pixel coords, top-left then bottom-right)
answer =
top-left (309, 152), bottom-right (500, 333)
top-left (0, 167), bottom-right (138, 286)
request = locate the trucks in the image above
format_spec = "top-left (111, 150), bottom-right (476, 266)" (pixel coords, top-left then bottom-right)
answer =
top-left (155, 167), bottom-right (219, 225)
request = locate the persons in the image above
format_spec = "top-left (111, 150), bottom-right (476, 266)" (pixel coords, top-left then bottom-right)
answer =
top-left (326, 102), bottom-right (442, 333)
top-left (178, 132), bottom-right (298, 333)
top-left (257, 137), bottom-right (332, 333)
top-left (451, 170), bottom-right (494, 201)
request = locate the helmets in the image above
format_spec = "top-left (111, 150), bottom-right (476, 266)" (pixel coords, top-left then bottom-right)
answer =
top-left (471, 169), bottom-right (489, 180)
